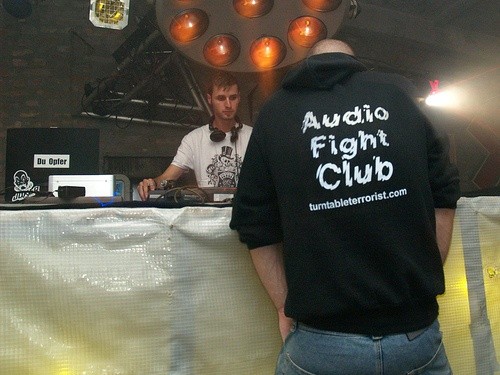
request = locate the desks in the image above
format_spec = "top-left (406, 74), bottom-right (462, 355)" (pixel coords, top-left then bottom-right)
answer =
top-left (1, 202), bottom-right (500, 374)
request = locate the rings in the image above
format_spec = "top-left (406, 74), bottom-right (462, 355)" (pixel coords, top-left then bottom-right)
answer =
top-left (148, 182), bottom-right (156, 186)
top-left (138, 183), bottom-right (143, 186)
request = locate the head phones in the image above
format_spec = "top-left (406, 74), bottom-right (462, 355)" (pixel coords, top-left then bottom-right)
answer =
top-left (208, 114), bottom-right (243, 143)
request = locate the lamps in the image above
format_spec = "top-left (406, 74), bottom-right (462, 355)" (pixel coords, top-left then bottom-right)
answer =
top-left (155, 0), bottom-right (349, 74)
top-left (88, 0), bottom-right (129, 31)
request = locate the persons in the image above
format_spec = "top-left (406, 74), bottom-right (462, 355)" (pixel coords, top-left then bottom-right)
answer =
top-left (136, 71), bottom-right (254, 203)
top-left (228, 38), bottom-right (462, 375)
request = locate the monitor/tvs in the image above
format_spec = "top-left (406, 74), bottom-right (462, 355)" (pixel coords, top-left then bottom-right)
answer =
top-left (6, 128), bottom-right (100, 201)
